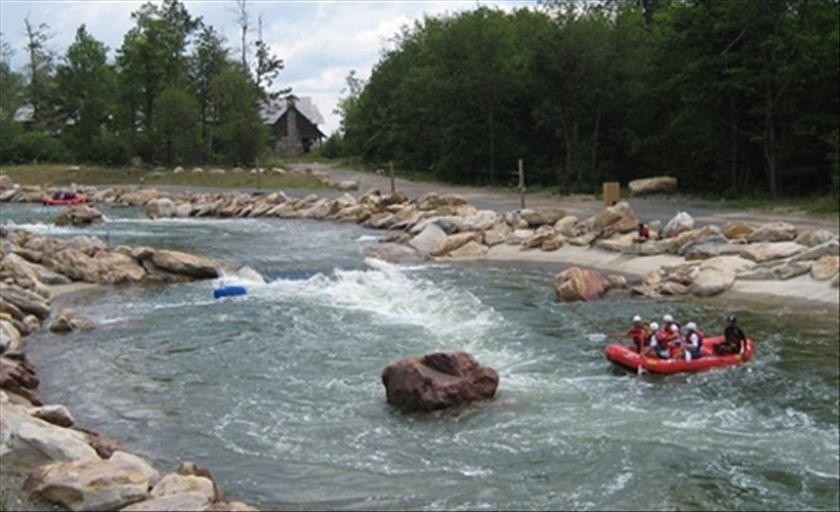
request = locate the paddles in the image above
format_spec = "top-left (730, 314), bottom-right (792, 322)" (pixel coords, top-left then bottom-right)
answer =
top-left (675, 330), bottom-right (692, 362)
top-left (586, 332), bottom-right (639, 343)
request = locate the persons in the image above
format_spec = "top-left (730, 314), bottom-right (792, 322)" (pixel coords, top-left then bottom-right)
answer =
top-left (673, 321), bottom-right (704, 358)
top-left (633, 223), bottom-right (650, 242)
top-left (713, 315), bottom-right (747, 356)
top-left (661, 313), bottom-right (681, 349)
top-left (640, 321), bottom-right (670, 359)
top-left (607, 314), bottom-right (652, 353)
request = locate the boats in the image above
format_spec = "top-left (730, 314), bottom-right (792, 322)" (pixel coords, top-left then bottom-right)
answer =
top-left (604, 333), bottom-right (755, 380)
top-left (42, 190), bottom-right (90, 205)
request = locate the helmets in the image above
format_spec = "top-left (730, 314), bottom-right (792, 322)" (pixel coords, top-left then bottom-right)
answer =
top-left (727, 315), bottom-right (737, 323)
top-left (663, 314), bottom-right (673, 322)
top-left (687, 322), bottom-right (697, 330)
top-left (649, 322), bottom-right (659, 330)
top-left (634, 315), bottom-right (640, 322)
